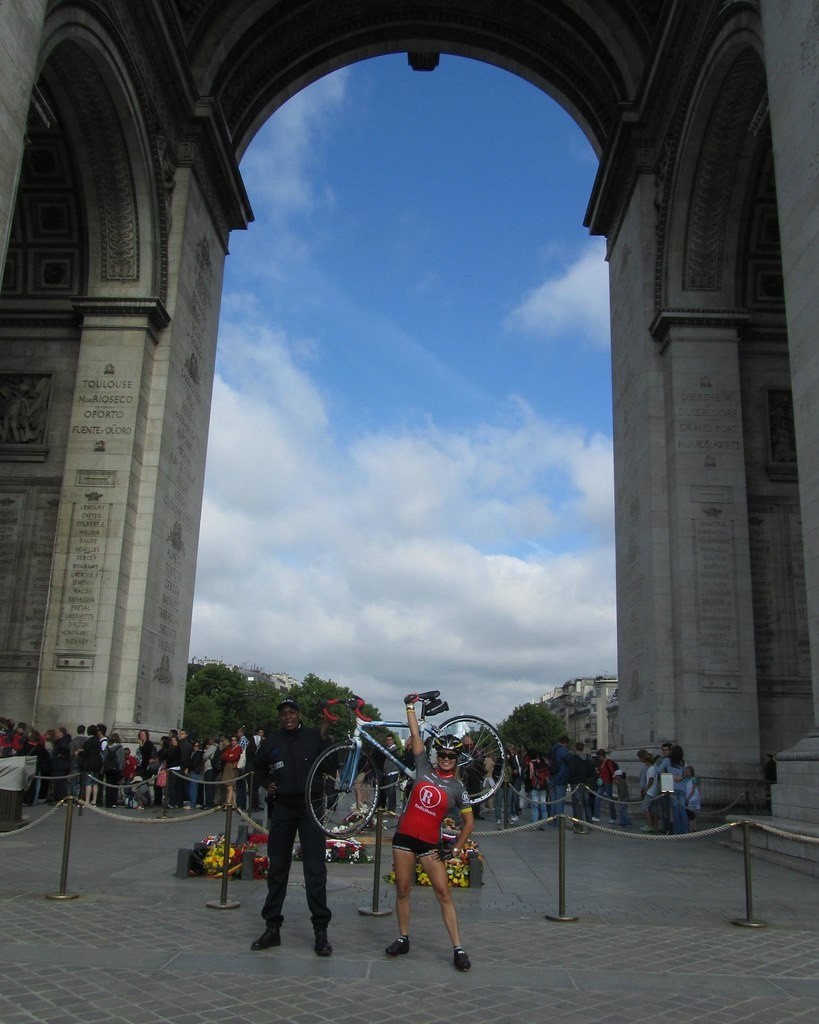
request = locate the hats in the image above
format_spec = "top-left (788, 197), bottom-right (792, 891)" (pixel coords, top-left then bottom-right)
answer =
top-left (130, 776), bottom-right (143, 784)
top-left (276, 697), bottom-right (299, 710)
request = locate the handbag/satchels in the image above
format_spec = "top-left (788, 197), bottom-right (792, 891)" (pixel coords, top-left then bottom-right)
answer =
top-left (212, 753), bottom-right (224, 771)
top-left (237, 746), bottom-right (247, 772)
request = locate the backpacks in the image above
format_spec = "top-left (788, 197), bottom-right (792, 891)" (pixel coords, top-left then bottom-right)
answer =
top-left (103, 745), bottom-right (122, 774)
top-left (532, 760), bottom-right (551, 790)
top-left (544, 745), bottom-right (562, 775)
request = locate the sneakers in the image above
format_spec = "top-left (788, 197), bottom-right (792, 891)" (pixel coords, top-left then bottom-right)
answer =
top-left (453, 949), bottom-right (471, 971)
top-left (385, 939), bottom-right (410, 956)
top-left (607, 819), bottom-right (617, 824)
top-left (592, 816), bottom-right (600, 822)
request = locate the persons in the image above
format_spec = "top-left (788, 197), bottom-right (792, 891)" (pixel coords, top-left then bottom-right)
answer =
top-left (253, 699), bottom-right (339, 956)
top-left (384, 693), bottom-right (474, 972)
top-left (0, 717), bottom-right (698, 834)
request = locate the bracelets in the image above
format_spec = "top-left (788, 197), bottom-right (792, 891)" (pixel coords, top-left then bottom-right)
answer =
top-left (453, 847), bottom-right (458, 853)
top-left (407, 710), bottom-right (415, 713)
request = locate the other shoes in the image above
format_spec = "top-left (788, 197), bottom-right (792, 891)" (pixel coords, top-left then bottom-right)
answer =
top-left (113, 802), bottom-right (265, 812)
top-left (63, 801), bottom-right (95, 806)
top-left (641, 824), bottom-right (672, 834)
top-left (497, 815), bottom-right (518, 825)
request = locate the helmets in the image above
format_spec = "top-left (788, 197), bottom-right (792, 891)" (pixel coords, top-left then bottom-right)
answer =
top-left (433, 734), bottom-right (464, 754)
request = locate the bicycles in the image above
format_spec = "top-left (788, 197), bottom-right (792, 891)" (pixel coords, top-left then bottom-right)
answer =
top-left (304, 690), bottom-right (509, 840)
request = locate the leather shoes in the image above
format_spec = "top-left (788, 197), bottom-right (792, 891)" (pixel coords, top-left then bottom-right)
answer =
top-left (251, 926), bottom-right (282, 950)
top-left (314, 930), bottom-right (332, 955)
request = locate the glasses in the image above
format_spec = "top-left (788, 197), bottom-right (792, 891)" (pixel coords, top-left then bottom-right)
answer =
top-left (193, 744), bottom-right (199, 747)
top-left (662, 748), bottom-right (669, 751)
top-left (437, 752), bottom-right (458, 760)
top-left (230, 740), bottom-right (237, 741)
